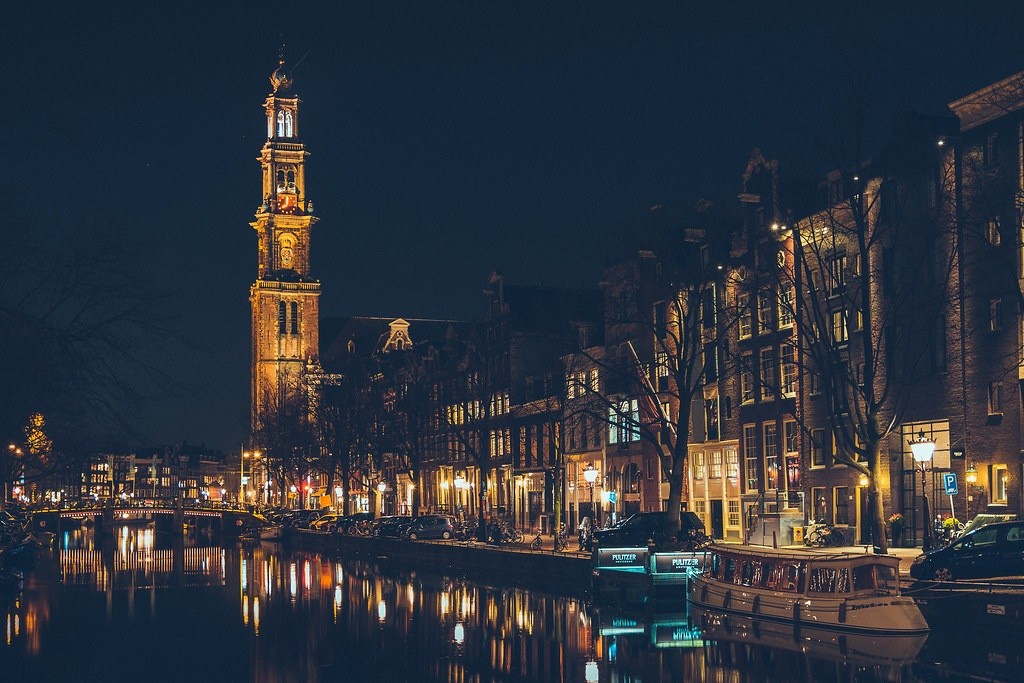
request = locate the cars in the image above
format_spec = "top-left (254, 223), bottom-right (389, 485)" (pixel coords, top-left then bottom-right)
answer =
top-left (587, 511), bottom-right (705, 547)
top-left (290, 509), bottom-right (458, 541)
top-left (909, 519), bottom-right (1024, 582)
top-left (0, 502), bottom-right (30, 613)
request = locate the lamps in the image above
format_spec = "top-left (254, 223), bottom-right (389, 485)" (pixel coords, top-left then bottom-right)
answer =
top-left (859, 472), bottom-right (869, 491)
top-left (965, 462), bottom-right (984, 490)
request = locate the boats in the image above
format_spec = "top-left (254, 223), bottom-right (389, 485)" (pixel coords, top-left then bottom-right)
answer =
top-left (686, 601), bottom-right (930, 671)
top-left (685, 542), bottom-right (930, 667)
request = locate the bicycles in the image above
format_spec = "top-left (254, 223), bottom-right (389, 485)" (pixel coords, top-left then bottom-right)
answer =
top-left (347, 521), bottom-right (373, 536)
top-left (530, 528), bottom-right (542, 550)
top-left (555, 531), bottom-right (570, 551)
top-left (452, 519), bottom-right (525, 545)
top-left (810, 524), bottom-right (846, 548)
top-left (670, 532), bottom-right (714, 552)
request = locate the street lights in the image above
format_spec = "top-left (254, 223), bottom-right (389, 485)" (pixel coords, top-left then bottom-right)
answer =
top-left (518, 475), bottom-right (527, 533)
top-left (906, 428), bottom-right (937, 547)
top-left (201, 474), bottom-right (492, 509)
top-left (581, 461), bottom-right (599, 530)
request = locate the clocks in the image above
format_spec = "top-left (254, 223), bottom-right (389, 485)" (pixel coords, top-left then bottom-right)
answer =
top-left (278, 195), bottom-right (297, 214)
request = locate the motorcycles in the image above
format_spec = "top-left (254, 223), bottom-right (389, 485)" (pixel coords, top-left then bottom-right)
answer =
top-left (576, 517), bottom-right (592, 552)
